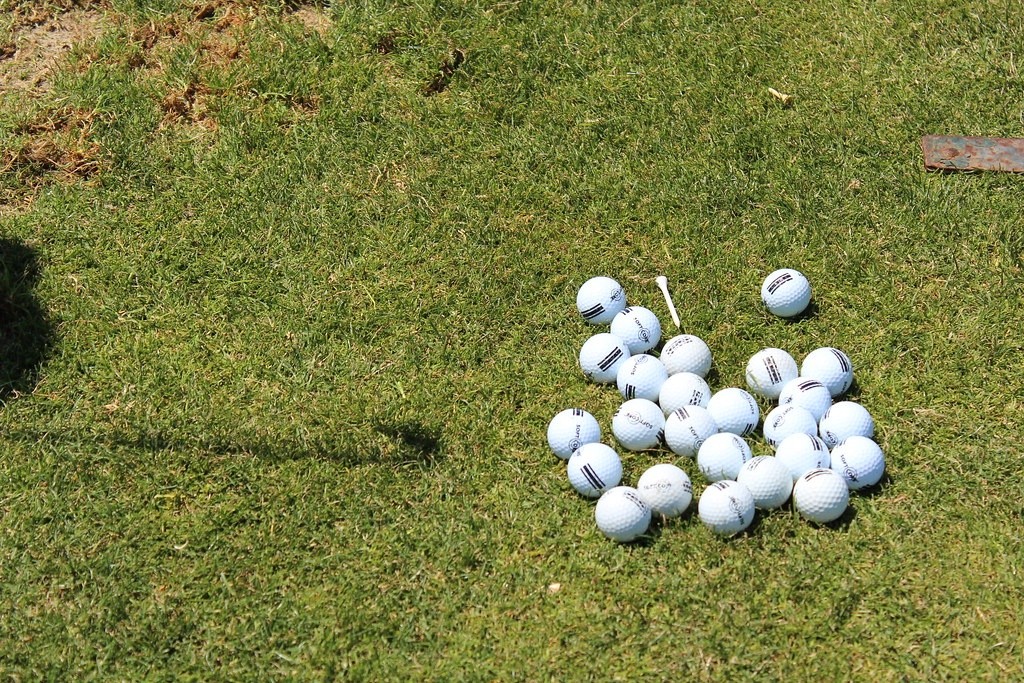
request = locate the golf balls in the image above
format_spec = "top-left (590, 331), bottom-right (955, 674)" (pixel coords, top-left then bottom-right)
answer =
top-left (548, 267), bottom-right (886, 541)
